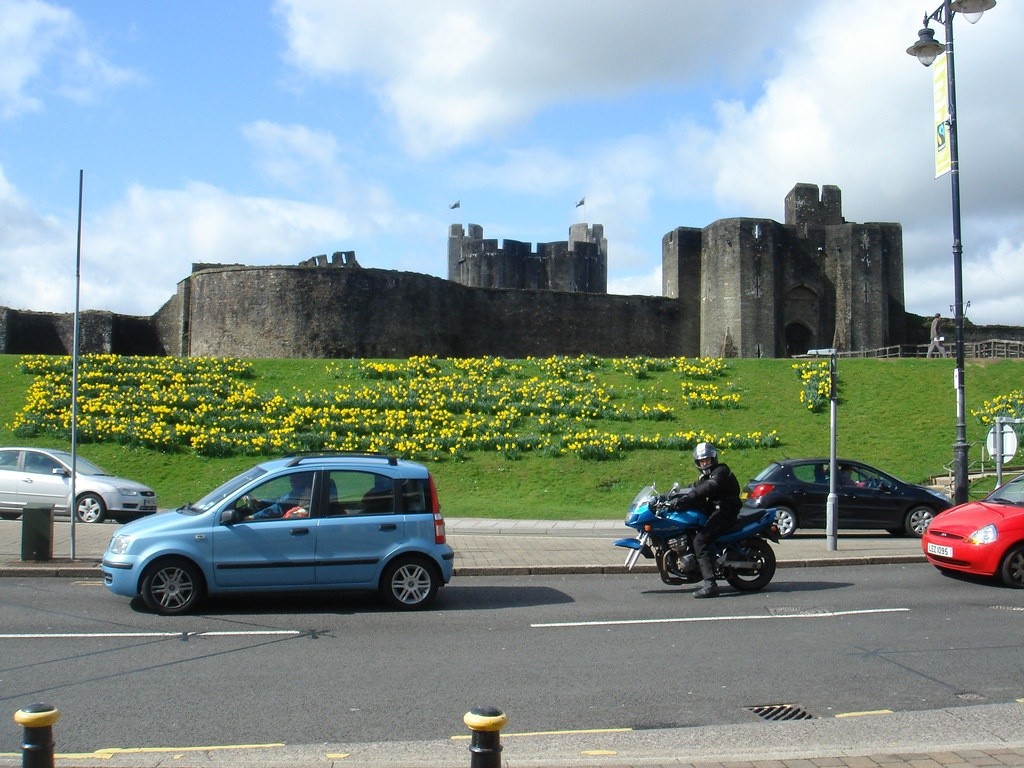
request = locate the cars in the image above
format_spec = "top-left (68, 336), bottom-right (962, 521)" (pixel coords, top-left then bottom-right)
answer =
top-left (922, 471), bottom-right (1024, 589)
top-left (1, 445), bottom-right (158, 524)
top-left (100, 449), bottom-right (457, 607)
top-left (740, 458), bottom-right (955, 536)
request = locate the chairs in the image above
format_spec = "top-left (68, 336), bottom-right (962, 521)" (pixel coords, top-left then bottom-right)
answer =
top-left (329, 479), bottom-right (344, 515)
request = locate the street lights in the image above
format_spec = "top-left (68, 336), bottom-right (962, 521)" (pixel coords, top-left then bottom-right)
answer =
top-left (906, 1), bottom-right (999, 507)
top-left (809, 349), bottom-right (837, 551)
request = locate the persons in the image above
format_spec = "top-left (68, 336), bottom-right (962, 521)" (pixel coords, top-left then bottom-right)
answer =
top-left (668, 443), bottom-right (743, 598)
top-left (244, 473), bottom-right (305, 521)
top-left (840, 466), bottom-right (870, 486)
top-left (926, 313), bottom-right (948, 358)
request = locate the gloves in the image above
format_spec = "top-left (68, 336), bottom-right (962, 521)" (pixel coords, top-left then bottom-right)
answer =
top-left (665, 491), bottom-right (678, 502)
top-left (669, 498), bottom-right (687, 512)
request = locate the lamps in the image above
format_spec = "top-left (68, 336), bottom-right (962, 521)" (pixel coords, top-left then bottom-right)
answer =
top-left (836, 244), bottom-right (839, 250)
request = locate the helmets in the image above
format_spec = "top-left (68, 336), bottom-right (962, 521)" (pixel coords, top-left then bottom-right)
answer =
top-left (693, 442), bottom-right (718, 476)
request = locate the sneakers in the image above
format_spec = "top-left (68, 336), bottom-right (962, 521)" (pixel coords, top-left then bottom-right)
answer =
top-left (691, 581), bottom-right (719, 598)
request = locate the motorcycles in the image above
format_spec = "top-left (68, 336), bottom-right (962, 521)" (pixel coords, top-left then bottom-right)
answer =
top-left (612, 480), bottom-right (781, 592)
